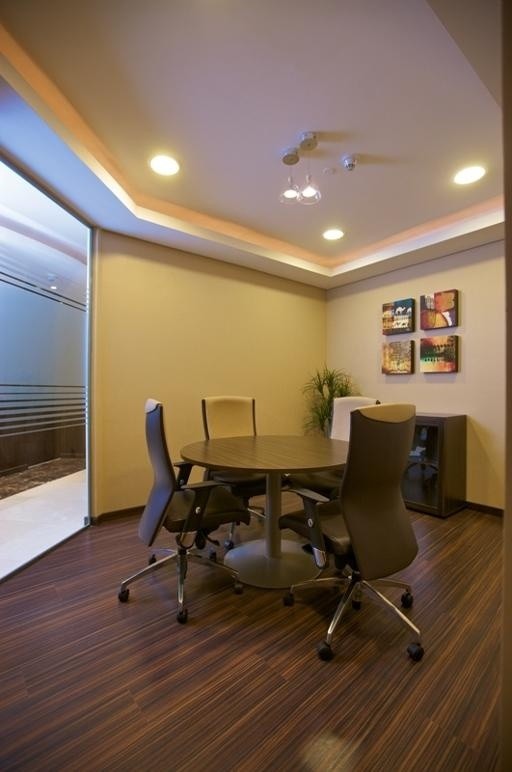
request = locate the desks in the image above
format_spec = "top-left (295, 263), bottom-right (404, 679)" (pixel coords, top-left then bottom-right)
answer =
top-left (179, 435), bottom-right (349, 590)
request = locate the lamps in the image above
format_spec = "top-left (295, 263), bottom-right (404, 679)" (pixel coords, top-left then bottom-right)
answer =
top-left (281, 131), bottom-right (321, 205)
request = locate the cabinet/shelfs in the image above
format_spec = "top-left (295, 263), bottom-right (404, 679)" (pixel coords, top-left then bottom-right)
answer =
top-left (400, 412), bottom-right (467, 518)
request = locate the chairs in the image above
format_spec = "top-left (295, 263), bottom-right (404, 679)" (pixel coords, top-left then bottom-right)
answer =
top-left (283, 403), bottom-right (425, 661)
top-left (199, 396), bottom-right (265, 551)
top-left (119, 395), bottom-right (252, 624)
